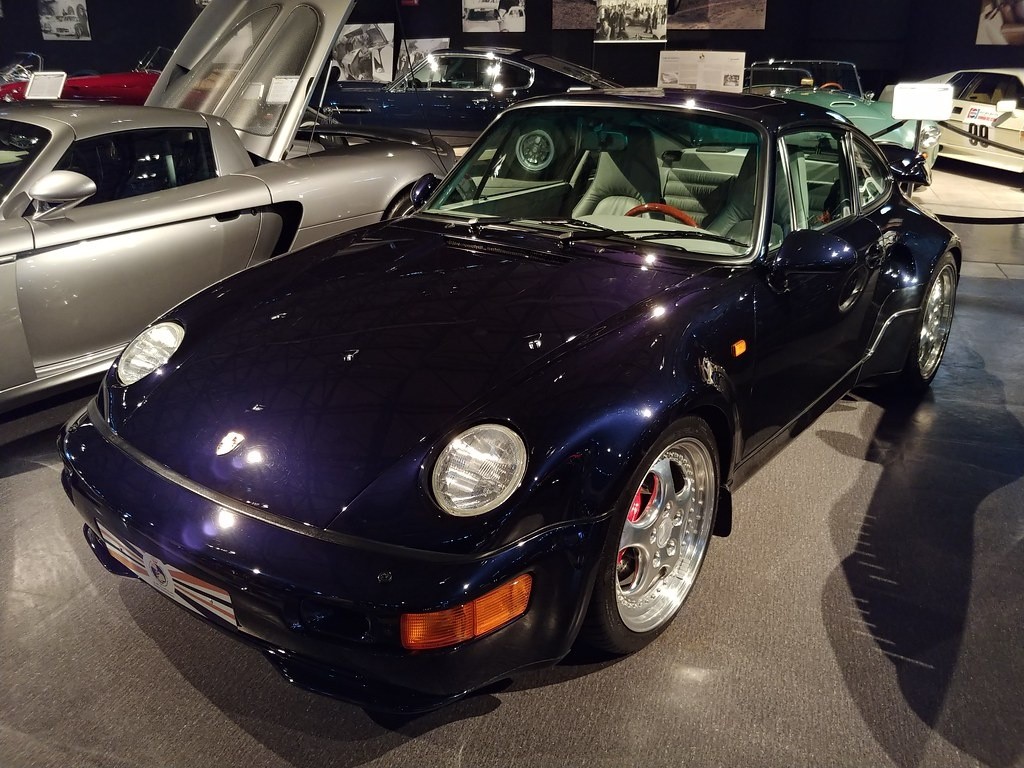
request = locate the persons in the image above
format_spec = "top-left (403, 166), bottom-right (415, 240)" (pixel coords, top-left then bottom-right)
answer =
top-left (365, 34), bottom-right (373, 47)
top-left (77, 5), bottom-right (90, 38)
top-left (464, 8), bottom-right (468, 19)
top-left (359, 46), bottom-right (384, 78)
top-left (361, 32), bottom-right (368, 48)
top-left (397, 49), bottom-right (435, 80)
top-left (596, 0), bottom-right (667, 42)
top-left (62, 8), bottom-right (67, 16)
top-left (337, 37), bottom-right (347, 65)
top-left (487, 9), bottom-right (497, 21)
top-left (519, 10), bottom-right (523, 16)
top-left (49, 8), bottom-right (55, 16)
top-left (511, 9), bottom-right (516, 17)
top-left (68, 7), bottom-right (75, 15)
top-left (41, 11), bottom-right (47, 30)
top-left (345, 34), bottom-right (354, 56)
top-left (351, 36), bottom-right (364, 51)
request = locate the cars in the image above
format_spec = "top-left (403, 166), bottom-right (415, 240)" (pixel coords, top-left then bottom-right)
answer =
top-left (0, 2), bottom-right (1022, 726)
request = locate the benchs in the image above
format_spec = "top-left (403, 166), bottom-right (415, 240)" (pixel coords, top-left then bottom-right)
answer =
top-left (658, 146), bottom-right (871, 244)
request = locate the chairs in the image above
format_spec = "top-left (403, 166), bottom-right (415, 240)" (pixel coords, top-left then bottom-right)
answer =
top-left (570, 126), bottom-right (664, 221)
top-left (703, 142), bottom-right (794, 253)
